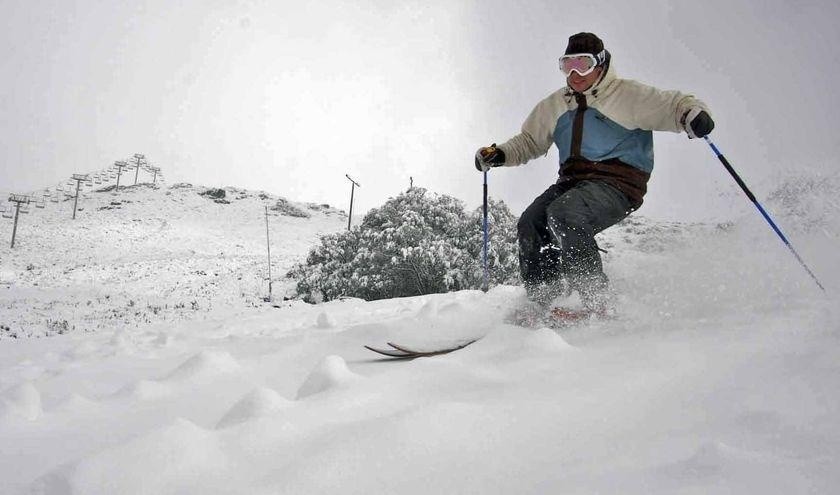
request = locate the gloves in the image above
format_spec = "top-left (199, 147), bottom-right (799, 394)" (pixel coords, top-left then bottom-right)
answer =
top-left (472, 142), bottom-right (506, 172)
top-left (678, 107), bottom-right (715, 142)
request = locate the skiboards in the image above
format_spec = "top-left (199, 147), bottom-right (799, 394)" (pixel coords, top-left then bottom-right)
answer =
top-left (363, 293), bottom-right (618, 358)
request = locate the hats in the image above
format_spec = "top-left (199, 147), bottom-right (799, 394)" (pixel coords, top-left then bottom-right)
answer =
top-left (564, 32), bottom-right (605, 55)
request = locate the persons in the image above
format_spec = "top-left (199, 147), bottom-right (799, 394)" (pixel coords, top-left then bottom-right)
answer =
top-left (473, 31), bottom-right (717, 327)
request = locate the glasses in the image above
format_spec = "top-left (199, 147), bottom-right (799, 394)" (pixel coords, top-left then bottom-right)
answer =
top-left (558, 50), bottom-right (608, 77)
